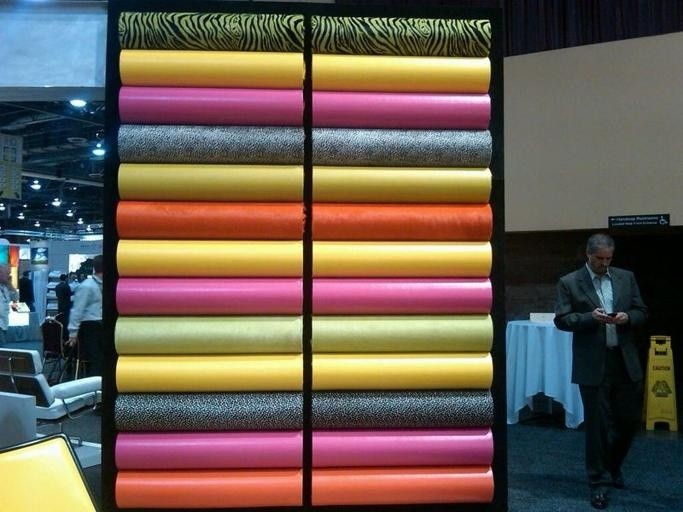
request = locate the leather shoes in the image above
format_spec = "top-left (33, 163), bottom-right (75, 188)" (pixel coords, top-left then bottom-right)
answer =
top-left (590, 466), bottom-right (623, 509)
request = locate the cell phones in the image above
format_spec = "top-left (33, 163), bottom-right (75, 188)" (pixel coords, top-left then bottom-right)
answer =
top-left (606, 313), bottom-right (618, 317)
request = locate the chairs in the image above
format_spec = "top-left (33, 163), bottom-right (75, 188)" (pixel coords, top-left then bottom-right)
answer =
top-left (0, 432), bottom-right (100, 511)
top-left (1, 348), bottom-right (103, 447)
top-left (39, 316), bottom-right (73, 383)
top-left (55, 312), bottom-right (69, 342)
top-left (58, 337), bottom-right (88, 384)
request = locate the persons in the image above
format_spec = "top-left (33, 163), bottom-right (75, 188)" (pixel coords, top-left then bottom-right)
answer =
top-left (553, 234), bottom-right (650, 509)
top-left (0, 254), bottom-right (105, 379)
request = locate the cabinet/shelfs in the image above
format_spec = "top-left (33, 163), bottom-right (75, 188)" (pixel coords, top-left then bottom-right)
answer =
top-left (45, 273), bottom-right (69, 317)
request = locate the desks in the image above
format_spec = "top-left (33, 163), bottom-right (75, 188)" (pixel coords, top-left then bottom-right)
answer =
top-left (506, 320), bottom-right (586, 429)
